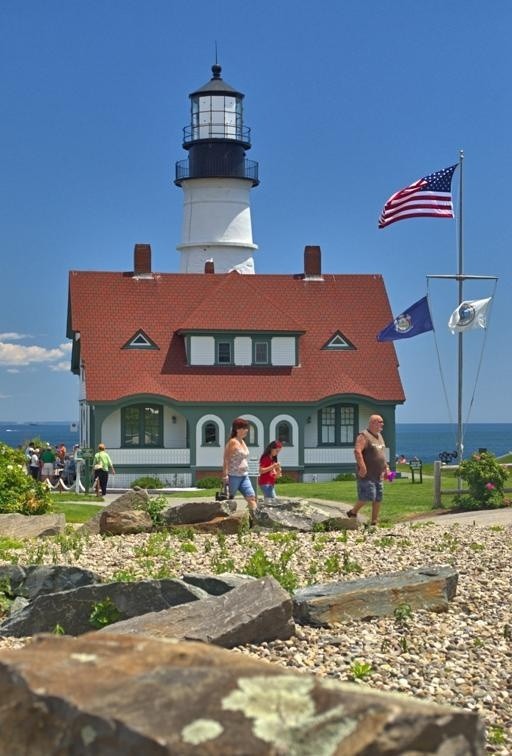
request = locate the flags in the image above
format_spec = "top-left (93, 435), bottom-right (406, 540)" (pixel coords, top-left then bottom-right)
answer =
top-left (377, 160), bottom-right (462, 228)
top-left (447, 293), bottom-right (492, 334)
top-left (376, 293), bottom-right (435, 342)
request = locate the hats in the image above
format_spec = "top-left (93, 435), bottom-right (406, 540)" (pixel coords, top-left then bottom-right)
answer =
top-left (33, 449), bottom-right (39, 453)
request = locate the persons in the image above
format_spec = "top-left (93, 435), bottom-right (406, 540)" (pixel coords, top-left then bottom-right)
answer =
top-left (258, 440), bottom-right (283, 498)
top-left (25, 438), bottom-right (81, 486)
top-left (345, 413), bottom-right (392, 526)
top-left (395, 454), bottom-right (400, 464)
top-left (399, 454), bottom-right (407, 464)
top-left (221, 418), bottom-right (257, 516)
top-left (92, 443), bottom-right (115, 496)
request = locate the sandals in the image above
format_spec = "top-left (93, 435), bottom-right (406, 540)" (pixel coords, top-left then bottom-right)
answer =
top-left (347, 510), bottom-right (356, 518)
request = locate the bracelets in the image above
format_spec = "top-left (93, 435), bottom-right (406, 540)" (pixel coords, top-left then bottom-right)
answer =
top-left (221, 475), bottom-right (229, 480)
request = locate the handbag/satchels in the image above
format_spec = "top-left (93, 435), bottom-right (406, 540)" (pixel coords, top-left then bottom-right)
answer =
top-left (94, 464), bottom-right (102, 470)
top-left (270, 469), bottom-right (282, 478)
top-left (215, 492), bottom-right (234, 501)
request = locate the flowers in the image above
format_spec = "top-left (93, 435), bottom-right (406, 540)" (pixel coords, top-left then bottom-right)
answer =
top-left (384, 468), bottom-right (397, 482)
